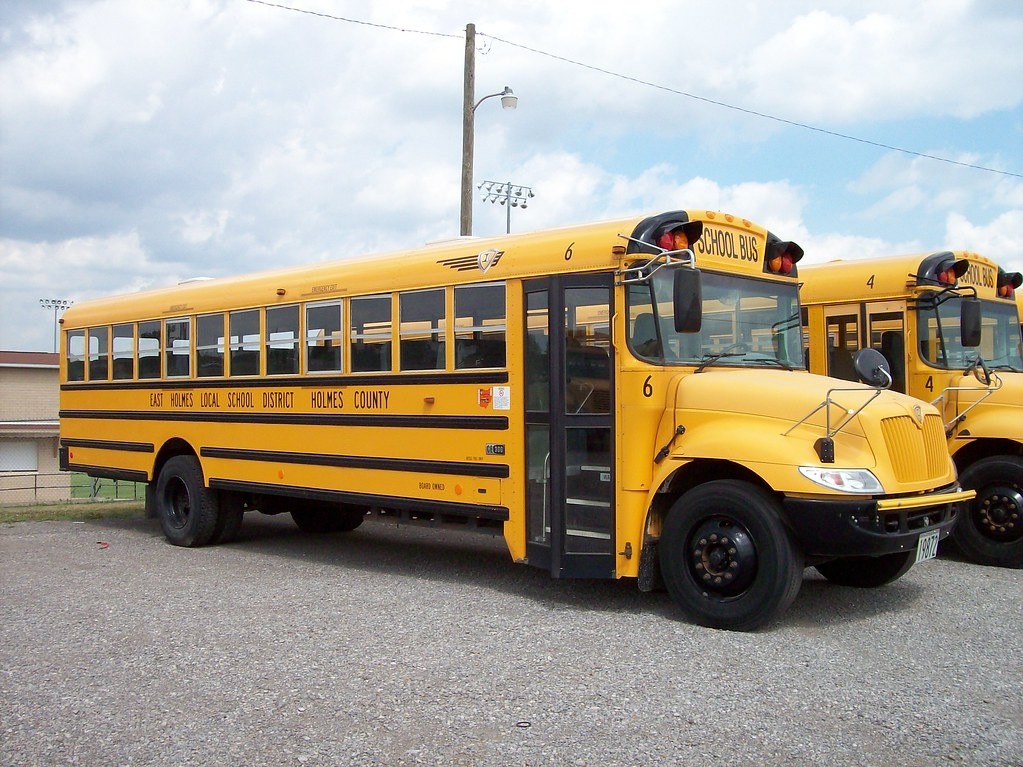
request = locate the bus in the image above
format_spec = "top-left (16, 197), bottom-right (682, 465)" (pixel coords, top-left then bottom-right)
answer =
top-left (57, 209), bottom-right (976, 635)
top-left (314, 249), bottom-right (1022, 568)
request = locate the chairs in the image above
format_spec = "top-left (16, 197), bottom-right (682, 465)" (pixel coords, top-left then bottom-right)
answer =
top-left (805, 343), bottom-right (858, 384)
top-left (631, 313), bottom-right (678, 361)
top-left (881, 332), bottom-right (905, 381)
top-left (69, 338), bottom-right (505, 381)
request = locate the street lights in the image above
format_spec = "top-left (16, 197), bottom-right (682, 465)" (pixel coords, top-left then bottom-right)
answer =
top-left (459, 23), bottom-right (518, 238)
